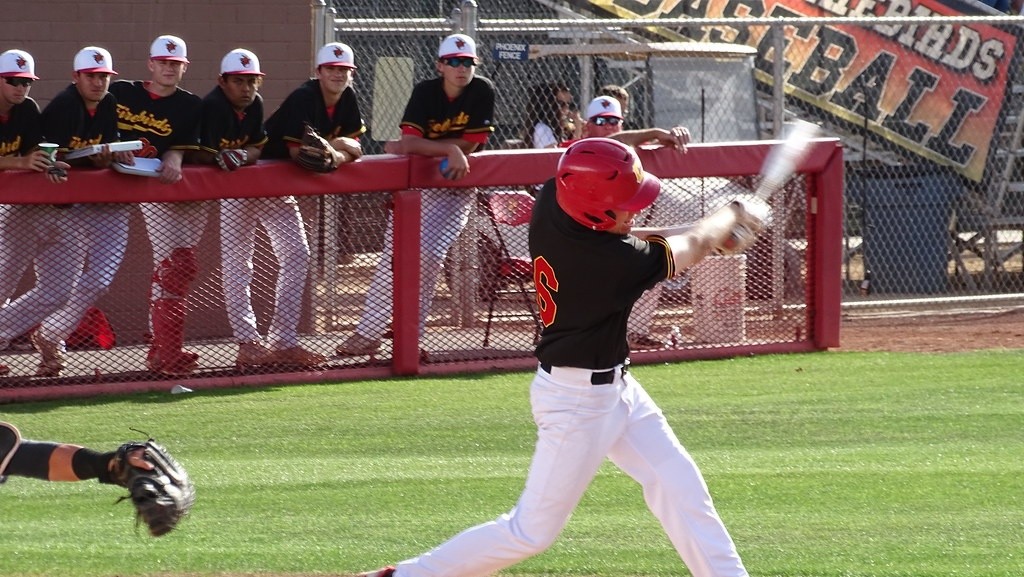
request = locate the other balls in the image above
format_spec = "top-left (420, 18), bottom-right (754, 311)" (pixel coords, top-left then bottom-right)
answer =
top-left (440, 158), bottom-right (449, 179)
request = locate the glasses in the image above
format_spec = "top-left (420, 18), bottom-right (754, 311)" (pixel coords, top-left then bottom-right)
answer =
top-left (1, 77), bottom-right (33, 87)
top-left (590, 116), bottom-right (621, 126)
top-left (557, 100), bottom-right (576, 110)
top-left (439, 57), bottom-right (474, 68)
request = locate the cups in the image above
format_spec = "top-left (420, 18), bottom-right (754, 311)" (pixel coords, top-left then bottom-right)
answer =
top-left (37, 143), bottom-right (60, 166)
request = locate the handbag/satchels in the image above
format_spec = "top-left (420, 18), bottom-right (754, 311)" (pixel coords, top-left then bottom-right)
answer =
top-left (27, 305), bottom-right (115, 351)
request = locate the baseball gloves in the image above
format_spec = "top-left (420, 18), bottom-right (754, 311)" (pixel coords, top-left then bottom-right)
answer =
top-left (293, 123), bottom-right (338, 174)
top-left (115, 439), bottom-right (195, 537)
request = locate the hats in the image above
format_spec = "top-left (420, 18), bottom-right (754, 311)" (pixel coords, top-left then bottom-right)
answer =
top-left (0, 49), bottom-right (39, 80)
top-left (149, 34), bottom-right (190, 64)
top-left (73, 46), bottom-right (118, 76)
top-left (586, 96), bottom-right (623, 121)
top-left (438, 34), bottom-right (479, 60)
top-left (316, 42), bottom-right (357, 69)
top-left (219, 48), bottom-right (266, 76)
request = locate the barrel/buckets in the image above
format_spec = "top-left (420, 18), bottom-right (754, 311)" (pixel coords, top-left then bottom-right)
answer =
top-left (862, 166), bottom-right (956, 293)
top-left (690, 254), bottom-right (746, 344)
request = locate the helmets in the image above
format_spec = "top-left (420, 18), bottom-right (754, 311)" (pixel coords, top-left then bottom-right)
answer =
top-left (555, 137), bottom-right (661, 230)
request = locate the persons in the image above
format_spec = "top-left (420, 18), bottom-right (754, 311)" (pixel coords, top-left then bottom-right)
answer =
top-left (599, 85), bottom-right (641, 131)
top-left (359, 137), bottom-right (764, 577)
top-left (139, 48), bottom-right (278, 370)
top-left (523, 83), bottom-right (588, 195)
top-left (558, 95), bottom-right (692, 350)
top-left (30, 34), bottom-right (202, 375)
top-left (0, 421), bottom-right (198, 537)
top-left (219, 42), bottom-right (366, 365)
top-left (334, 34), bottom-right (496, 354)
top-left (0, 49), bottom-right (71, 183)
top-left (0, 46), bottom-right (120, 369)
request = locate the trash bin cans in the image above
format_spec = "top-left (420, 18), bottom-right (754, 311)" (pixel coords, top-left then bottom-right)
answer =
top-left (855, 165), bottom-right (956, 291)
top-left (691, 254), bottom-right (745, 343)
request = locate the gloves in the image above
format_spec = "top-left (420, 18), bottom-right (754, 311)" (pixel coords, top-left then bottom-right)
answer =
top-left (730, 197), bottom-right (774, 233)
top-left (215, 149), bottom-right (248, 170)
top-left (710, 224), bottom-right (756, 256)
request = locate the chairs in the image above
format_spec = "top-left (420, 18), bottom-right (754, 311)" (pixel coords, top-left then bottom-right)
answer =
top-left (478, 190), bottom-right (544, 347)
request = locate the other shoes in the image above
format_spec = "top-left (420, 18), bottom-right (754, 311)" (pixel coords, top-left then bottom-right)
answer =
top-left (31, 330), bottom-right (68, 369)
top-left (146, 347), bottom-right (199, 375)
top-left (626, 331), bottom-right (664, 350)
top-left (236, 342), bottom-right (281, 365)
top-left (0, 364), bottom-right (10, 374)
top-left (364, 566), bottom-right (395, 577)
top-left (336, 332), bottom-right (382, 356)
top-left (269, 345), bottom-right (330, 366)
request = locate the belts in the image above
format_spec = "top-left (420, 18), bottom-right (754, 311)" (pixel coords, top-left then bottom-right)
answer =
top-left (540, 361), bottom-right (630, 386)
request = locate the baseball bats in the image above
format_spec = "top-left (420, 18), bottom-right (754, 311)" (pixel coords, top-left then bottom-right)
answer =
top-left (719, 119), bottom-right (823, 250)
